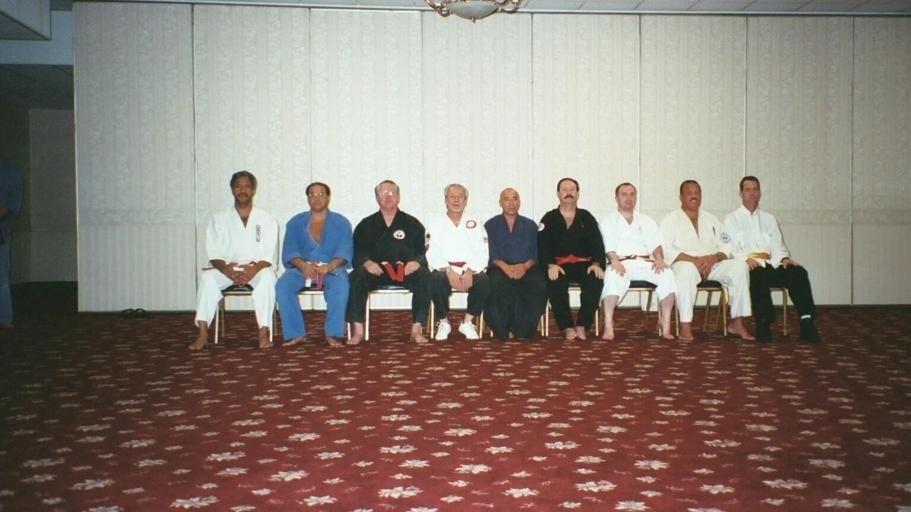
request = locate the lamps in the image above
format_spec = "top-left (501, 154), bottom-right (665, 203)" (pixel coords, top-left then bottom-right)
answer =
top-left (427, 0), bottom-right (518, 22)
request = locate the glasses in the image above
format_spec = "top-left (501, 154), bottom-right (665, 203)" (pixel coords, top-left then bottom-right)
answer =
top-left (308, 191), bottom-right (326, 197)
top-left (377, 190), bottom-right (395, 197)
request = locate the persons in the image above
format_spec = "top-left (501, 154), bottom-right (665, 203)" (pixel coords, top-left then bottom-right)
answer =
top-left (598, 183), bottom-right (677, 340)
top-left (723, 177), bottom-right (821, 343)
top-left (423, 184), bottom-right (490, 341)
top-left (661, 180), bottom-right (756, 341)
top-left (484, 188), bottom-right (548, 341)
top-left (188, 172), bottom-right (279, 351)
top-left (275, 182), bottom-right (354, 347)
top-left (345, 179), bottom-right (434, 345)
top-left (538, 178), bottom-right (605, 340)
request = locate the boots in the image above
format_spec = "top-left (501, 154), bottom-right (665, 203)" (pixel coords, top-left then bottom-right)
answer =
top-left (755, 320), bottom-right (774, 344)
top-left (798, 318), bottom-right (823, 344)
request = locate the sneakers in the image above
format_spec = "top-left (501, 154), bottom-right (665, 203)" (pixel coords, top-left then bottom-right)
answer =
top-left (458, 322), bottom-right (480, 339)
top-left (435, 321), bottom-right (451, 340)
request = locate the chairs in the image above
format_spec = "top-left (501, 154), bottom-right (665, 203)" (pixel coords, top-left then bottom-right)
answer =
top-left (216, 279), bottom-right (785, 343)
